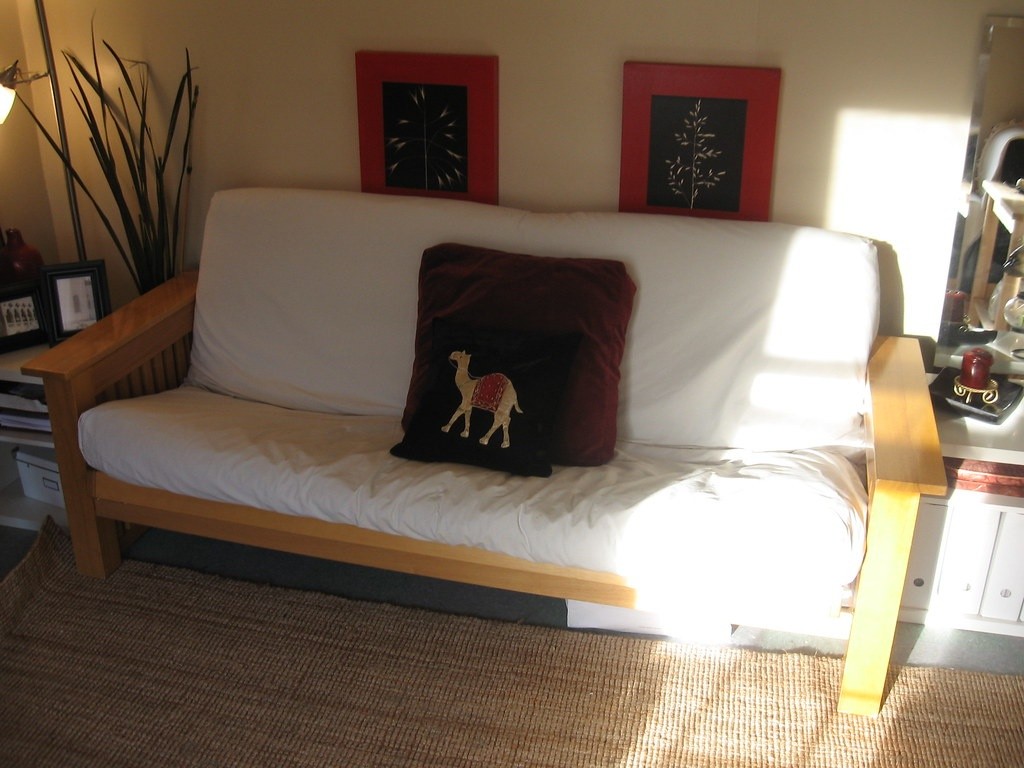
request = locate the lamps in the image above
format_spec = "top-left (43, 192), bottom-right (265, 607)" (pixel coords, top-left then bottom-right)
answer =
top-left (0, 59), bottom-right (87, 262)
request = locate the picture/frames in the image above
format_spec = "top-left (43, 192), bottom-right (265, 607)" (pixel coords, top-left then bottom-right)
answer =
top-left (0, 275), bottom-right (49, 352)
top-left (618, 62), bottom-right (781, 223)
top-left (39, 259), bottom-right (112, 347)
top-left (356, 50), bottom-right (499, 205)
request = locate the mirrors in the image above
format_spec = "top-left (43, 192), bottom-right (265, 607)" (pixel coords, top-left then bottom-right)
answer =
top-left (935, 17), bottom-right (1024, 374)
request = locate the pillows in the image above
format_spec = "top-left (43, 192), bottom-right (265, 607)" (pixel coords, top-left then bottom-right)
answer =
top-left (391, 317), bottom-right (585, 477)
top-left (401, 243), bottom-right (637, 466)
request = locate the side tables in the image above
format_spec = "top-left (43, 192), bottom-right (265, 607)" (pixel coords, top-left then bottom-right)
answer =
top-left (0, 345), bottom-right (69, 539)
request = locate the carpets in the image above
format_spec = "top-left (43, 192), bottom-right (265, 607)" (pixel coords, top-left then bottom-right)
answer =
top-left (1, 517), bottom-right (1024, 768)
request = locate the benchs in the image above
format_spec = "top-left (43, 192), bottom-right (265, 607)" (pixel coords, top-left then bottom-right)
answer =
top-left (42, 186), bottom-right (953, 718)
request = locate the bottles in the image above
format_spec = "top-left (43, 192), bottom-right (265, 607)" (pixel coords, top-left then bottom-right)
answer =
top-left (0, 228), bottom-right (43, 281)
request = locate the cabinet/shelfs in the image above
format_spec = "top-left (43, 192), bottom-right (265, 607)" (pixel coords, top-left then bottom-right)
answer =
top-left (895, 372), bottom-right (1023, 640)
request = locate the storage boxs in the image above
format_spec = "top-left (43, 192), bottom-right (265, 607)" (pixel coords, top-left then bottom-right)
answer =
top-left (13, 446), bottom-right (65, 509)
